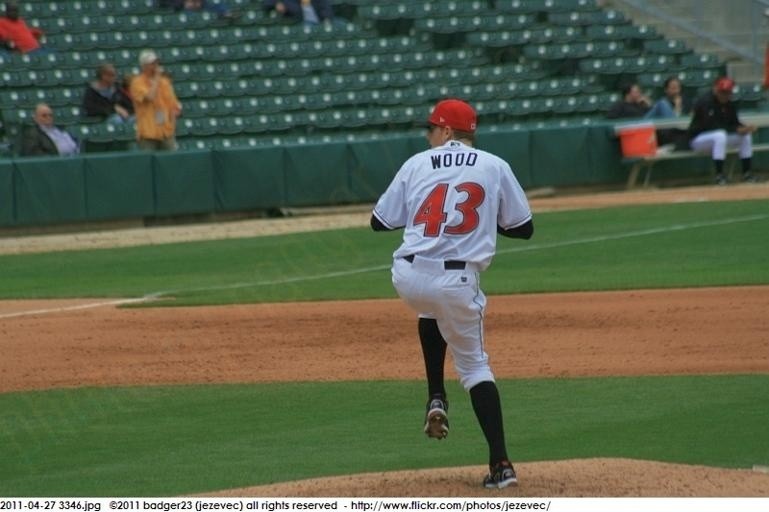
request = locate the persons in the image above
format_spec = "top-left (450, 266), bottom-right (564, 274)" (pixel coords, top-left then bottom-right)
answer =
top-left (761, 38), bottom-right (769, 90)
top-left (370, 99), bottom-right (534, 490)
top-left (23, 103), bottom-right (80, 155)
top-left (604, 82), bottom-right (652, 119)
top-left (686, 78), bottom-right (757, 187)
top-left (82, 48), bottom-right (183, 151)
top-left (641, 78), bottom-right (694, 146)
top-left (157, 0), bottom-right (335, 31)
top-left (0, 3), bottom-right (47, 56)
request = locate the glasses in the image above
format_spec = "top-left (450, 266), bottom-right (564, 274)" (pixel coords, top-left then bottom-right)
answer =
top-left (41, 111), bottom-right (54, 115)
top-left (428, 125), bottom-right (437, 133)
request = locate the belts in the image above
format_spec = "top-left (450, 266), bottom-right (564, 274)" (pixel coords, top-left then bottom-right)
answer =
top-left (403, 253), bottom-right (466, 272)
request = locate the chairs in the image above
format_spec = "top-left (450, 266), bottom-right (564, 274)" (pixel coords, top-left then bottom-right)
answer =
top-left (1, 0), bottom-right (769, 159)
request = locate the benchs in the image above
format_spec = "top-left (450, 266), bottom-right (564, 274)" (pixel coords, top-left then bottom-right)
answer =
top-left (620, 143), bottom-right (769, 190)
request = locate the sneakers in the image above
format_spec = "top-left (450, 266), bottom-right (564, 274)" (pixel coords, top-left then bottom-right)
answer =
top-left (484, 458), bottom-right (518, 489)
top-left (422, 396), bottom-right (453, 441)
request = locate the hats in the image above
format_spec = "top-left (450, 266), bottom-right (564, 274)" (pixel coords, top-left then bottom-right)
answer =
top-left (137, 48), bottom-right (160, 66)
top-left (428, 99), bottom-right (477, 133)
